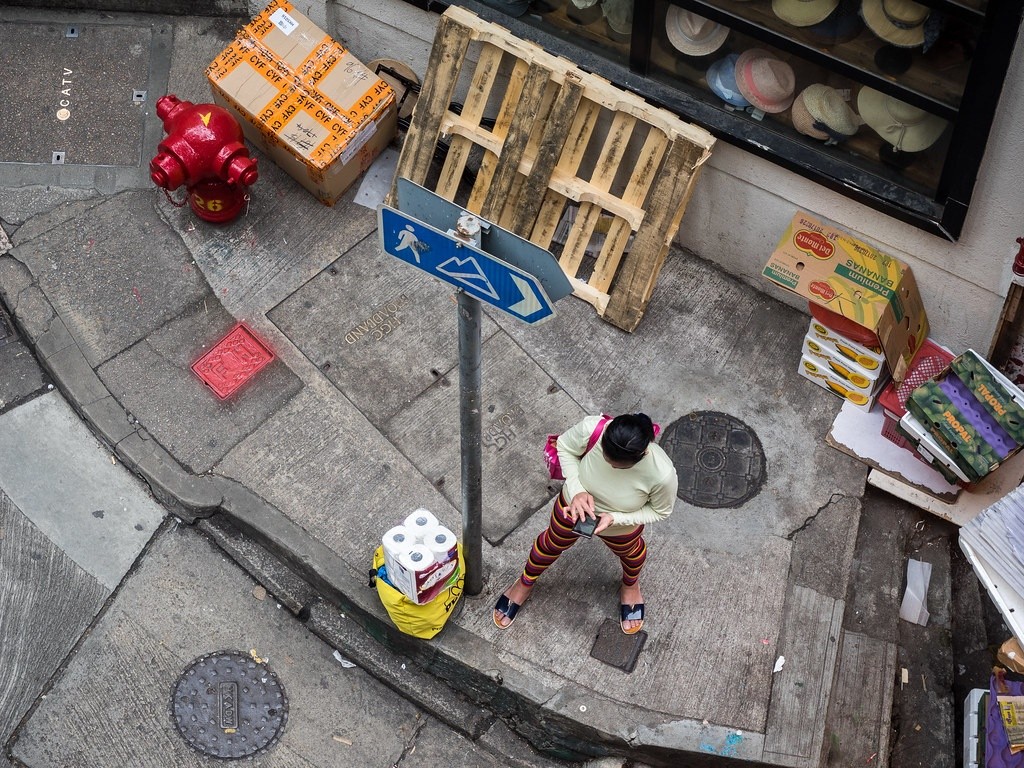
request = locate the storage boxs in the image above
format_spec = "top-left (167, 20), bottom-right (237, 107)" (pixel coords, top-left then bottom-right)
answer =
top-left (760, 211), bottom-right (1024, 487)
top-left (203, 0), bottom-right (396, 207)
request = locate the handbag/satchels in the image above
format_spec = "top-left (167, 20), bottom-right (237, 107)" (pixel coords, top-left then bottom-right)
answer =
top-left (368, 542), bottom-right (466, 639)
top-left (543, 415), bottom-right (661, 480)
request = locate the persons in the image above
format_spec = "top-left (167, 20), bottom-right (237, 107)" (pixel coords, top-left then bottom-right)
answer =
top-left (493, 412), bottom-right (678, 634)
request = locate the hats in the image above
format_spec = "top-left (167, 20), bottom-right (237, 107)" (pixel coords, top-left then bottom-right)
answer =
top-left (734, 48), bottom-right (795, 113)
top-left (792, 92), bottom-right (830, 140)
top-left (666, 4), bottom-right (730, 56)
top-left (802, 84), bottom-right (866, 136)
top-left (602, 0), bottom-right (632, 34)
top-left (706, 53), bottom-right (751, 106)
top-left (862, 0), bottom-right (928, 46)
top-left (773, 0), bottom-right (837, 26)
top-left (857, 86), bottom-right (949, 152)
top-left (572, 0), bottom-right (597, 9)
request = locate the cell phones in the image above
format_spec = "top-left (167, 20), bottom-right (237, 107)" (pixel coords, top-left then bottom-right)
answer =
top-left (572, 513), bottom-right (601, 538)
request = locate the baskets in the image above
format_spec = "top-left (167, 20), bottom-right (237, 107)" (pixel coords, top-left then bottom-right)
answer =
top-left (881, 418), bottom-right (981, 493)
top-left (879, 339), bottom-right (955, 417)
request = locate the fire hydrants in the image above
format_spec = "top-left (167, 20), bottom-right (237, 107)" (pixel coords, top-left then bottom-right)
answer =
top-left (146, 93), bottom-right (261, 229)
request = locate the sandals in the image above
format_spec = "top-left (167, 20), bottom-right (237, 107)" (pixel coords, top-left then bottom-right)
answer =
top-left (493, 578), bottom-right (533, 629)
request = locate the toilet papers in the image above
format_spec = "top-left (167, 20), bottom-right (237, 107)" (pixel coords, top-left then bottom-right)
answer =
top-left (381, 509), bottom-right (457, 605)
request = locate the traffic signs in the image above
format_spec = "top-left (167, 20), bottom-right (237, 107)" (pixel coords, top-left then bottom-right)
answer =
top-left (374, 204), bottom-right (560, 329)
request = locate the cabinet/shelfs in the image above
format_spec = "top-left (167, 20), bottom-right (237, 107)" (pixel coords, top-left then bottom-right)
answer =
top-left (462, 0), bottom-right (1024, 244)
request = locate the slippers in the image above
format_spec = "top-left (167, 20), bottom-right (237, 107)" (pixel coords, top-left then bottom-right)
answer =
top-left (620, 603), bottom-right (646, 634)
